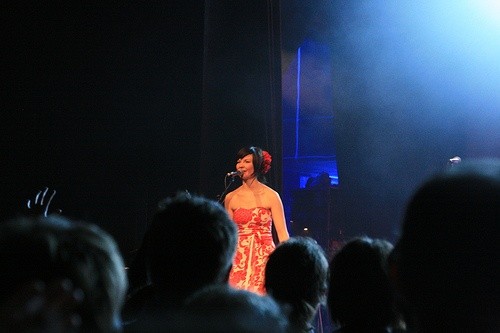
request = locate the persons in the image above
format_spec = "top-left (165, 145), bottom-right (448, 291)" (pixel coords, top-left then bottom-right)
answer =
top-left (326, 232), bottom-right (408, 332)
top-left (383, 172), bottom-right (500, 333)
top-left (263, 237), bottom-right (327, 333)
top-left (176, 283), bottom-right (289, 333)
top-left (127, 190), bottom-right (236, 333)
top-left (1, 214), bottom-right (126, 333)
top-left (24, 187), bottom-right (63, 218)
top-left (225, 144), bottom-right (290, 297)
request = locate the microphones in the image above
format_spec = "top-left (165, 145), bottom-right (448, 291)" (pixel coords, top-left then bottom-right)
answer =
top-left (449, 156), bottom-right (461, 163)
top-left (227, 169), bottom-right (244, 177)
